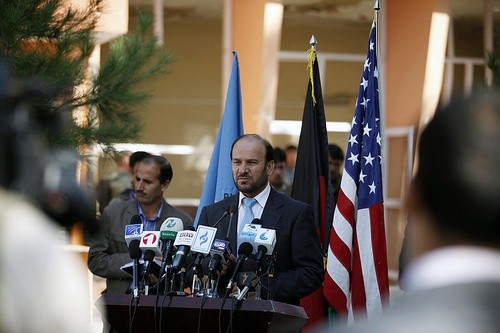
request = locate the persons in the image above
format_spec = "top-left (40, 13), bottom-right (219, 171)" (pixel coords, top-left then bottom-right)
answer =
top-left (269, 143), bottom-right (351, 267)
top-left (309, 90), bottom-right (500, 333)
top-left (186, 134), bottom-right (325, 306)
top-left (86, 152), bottom-right (194, 333)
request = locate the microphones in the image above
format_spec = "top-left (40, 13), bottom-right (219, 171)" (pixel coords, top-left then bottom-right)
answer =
top-left (124, 207), bottom-right (276, 306)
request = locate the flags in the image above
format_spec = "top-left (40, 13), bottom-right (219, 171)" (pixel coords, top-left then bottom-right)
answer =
top-left (193, 54), bottom-right (244, 231)
top-left (323, 20), bottom-right (389, 322)
top-left (291, 49), bottom-right (330, 333)
